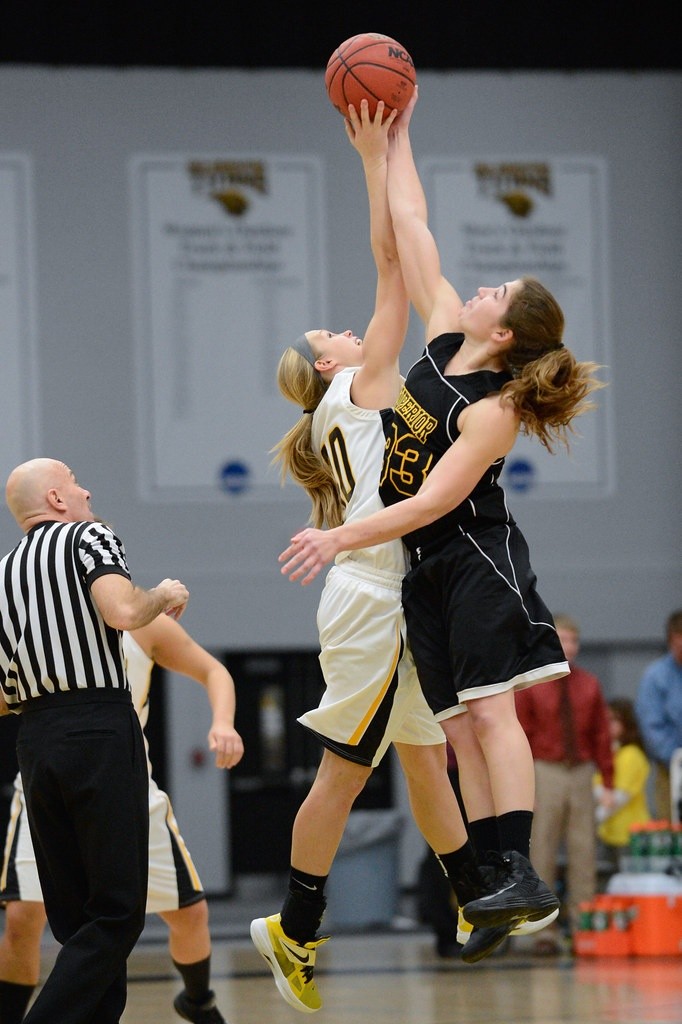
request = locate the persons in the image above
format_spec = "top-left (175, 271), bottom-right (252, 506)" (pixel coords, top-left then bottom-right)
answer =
top-left (513, 612), bottom-right (617, 956)
top-left (635, 608), bottom-right (682, 767)
top-left (592, 695), bottom-right (652, 869)
top-left (251, 82), bottom-right (599, 1013)
top-left (0, 456), bottom-right (243, 1023)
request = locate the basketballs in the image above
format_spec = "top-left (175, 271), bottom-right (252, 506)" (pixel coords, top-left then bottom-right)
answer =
top-left (324, 32), bottom-right (416, 125)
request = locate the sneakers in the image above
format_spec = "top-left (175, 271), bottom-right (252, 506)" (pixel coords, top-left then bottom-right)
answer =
top-left (171, 987), bottom-right (232, 1024)
top-left (457, 895), bottom-right (559, 945)
top-left (456, 848), bottom-right (561, 964)
top-left (250, 912), bottom-right (332, 1014)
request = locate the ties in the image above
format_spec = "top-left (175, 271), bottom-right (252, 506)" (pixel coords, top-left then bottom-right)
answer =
top-left (558, 675), bottom-right (582, 769)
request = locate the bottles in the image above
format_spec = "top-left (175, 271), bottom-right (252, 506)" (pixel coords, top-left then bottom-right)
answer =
top-left (622, 821), bottom-right (681, 872)
top-left (574, 900), bottom-right (628, 932)
